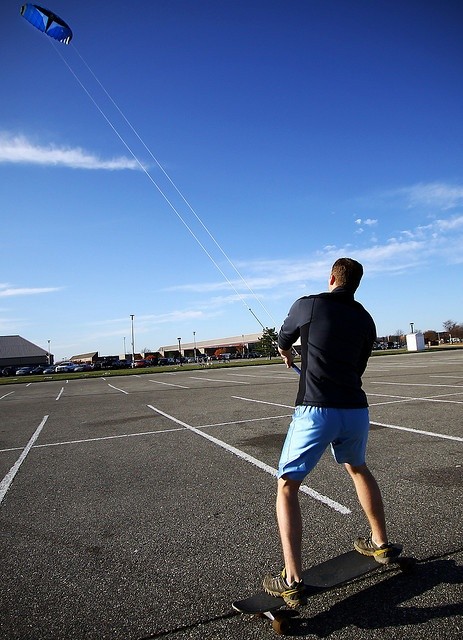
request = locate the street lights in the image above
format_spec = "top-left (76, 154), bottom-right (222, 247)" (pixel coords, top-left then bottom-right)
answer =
top-left (47, 340), bottom-right (50, 366)
top-left (193, 332), bottom-right (195, 348)
top-left (130, 314), bottom-right (135, 353)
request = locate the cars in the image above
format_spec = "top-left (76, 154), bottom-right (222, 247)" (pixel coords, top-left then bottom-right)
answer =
top-left (30, 368), bottom-right (43, 375)
top-left (43, 366), bottom-right (56, 374)
top-left (73, 364), bottom-right (87, 371)
top-left (16, 366), bottom-right (32, 376)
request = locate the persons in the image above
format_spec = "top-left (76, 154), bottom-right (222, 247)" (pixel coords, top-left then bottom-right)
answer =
top-left (262, 256), bottom-right (404, 609)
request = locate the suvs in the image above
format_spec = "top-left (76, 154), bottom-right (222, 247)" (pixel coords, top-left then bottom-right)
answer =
top-left (55, 362), bottom-right (75, 373)
top-left (131, 360), bottom-right (142, 368)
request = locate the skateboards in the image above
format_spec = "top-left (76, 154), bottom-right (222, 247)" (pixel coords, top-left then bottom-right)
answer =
top-left (231, 543), bottom-right (403, 633)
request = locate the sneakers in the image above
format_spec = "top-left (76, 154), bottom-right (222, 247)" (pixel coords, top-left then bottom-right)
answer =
top-left (353, 532), bottom-right (391, 564)
top-left (262, 568), bottom-right (304, 608)
